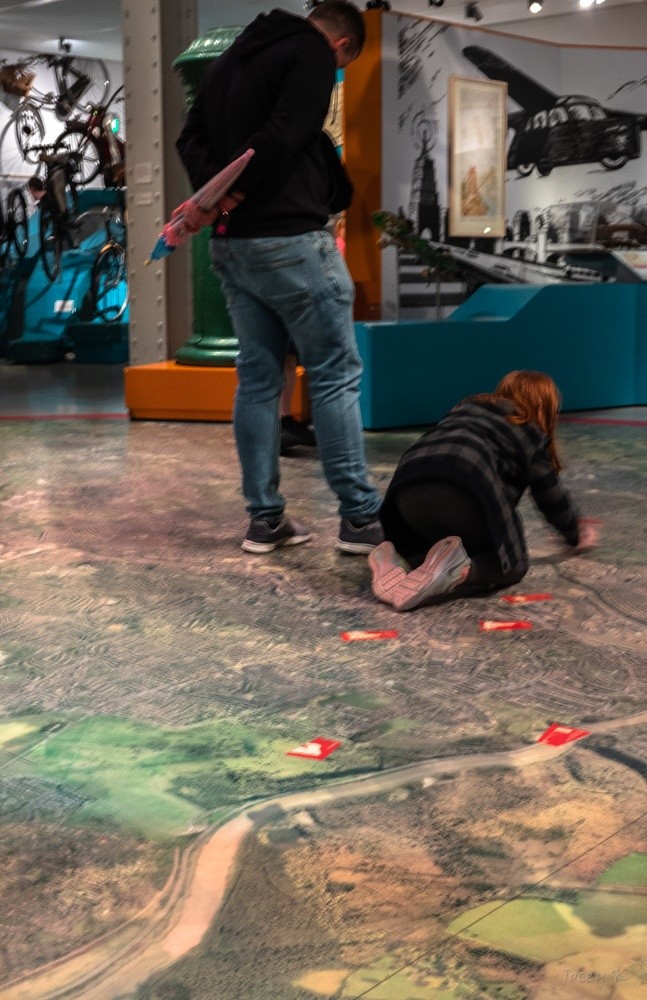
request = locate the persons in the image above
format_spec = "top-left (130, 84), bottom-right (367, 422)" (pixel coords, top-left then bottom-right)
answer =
top-left (176, 0), bottom-right (381, 555)
top-left (368, 371), bottom-right (594, 611)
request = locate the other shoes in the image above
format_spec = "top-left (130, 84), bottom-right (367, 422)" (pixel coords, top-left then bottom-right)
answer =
top-left (280, 415), bottom-right (318, 451)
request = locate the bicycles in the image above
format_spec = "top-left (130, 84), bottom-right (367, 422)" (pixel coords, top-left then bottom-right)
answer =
top-left (0, 49), bottom-right (129, 323)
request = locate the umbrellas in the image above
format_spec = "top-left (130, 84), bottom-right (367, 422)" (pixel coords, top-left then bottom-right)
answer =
top-left (141, 149), bottom-right (255, 267)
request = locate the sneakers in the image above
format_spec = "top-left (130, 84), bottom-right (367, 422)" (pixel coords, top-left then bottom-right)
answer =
top-left (392, 536), bottom-right (471, 611)
top-left (241, 515), bottom-right (313, 554)
top-left (367, 541), bottom-right (412, 604)
top-left (337, 517), bottom-right (385, 554)
